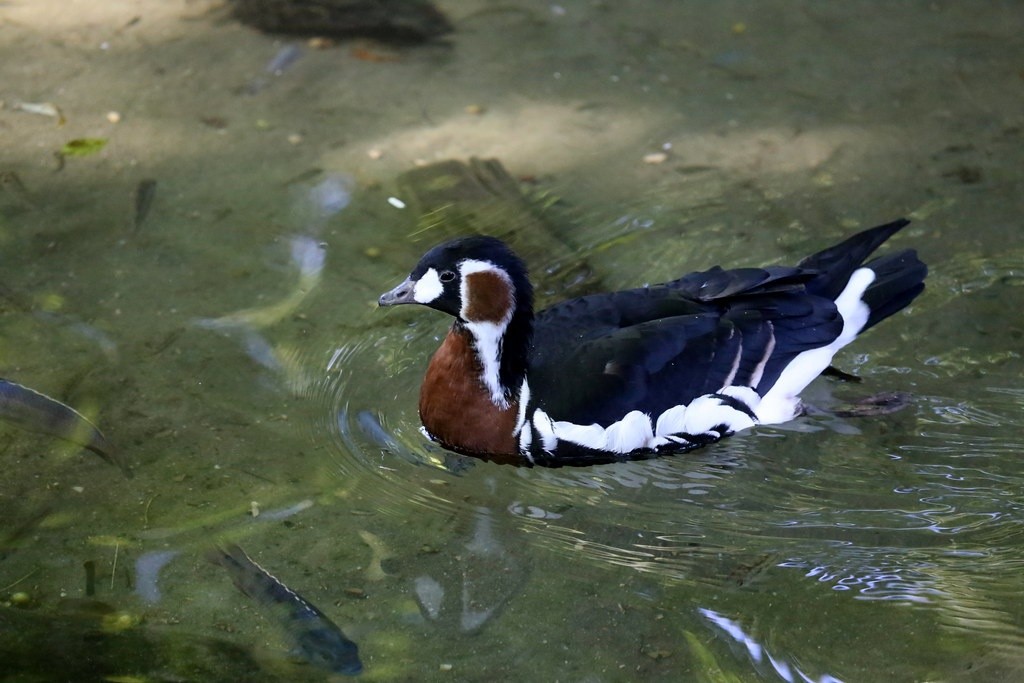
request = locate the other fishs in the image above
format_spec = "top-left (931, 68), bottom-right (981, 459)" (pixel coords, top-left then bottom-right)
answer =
top-left (204, 542), bottom-right (367, 679)
top-left (0, 377), bottom-right (133, 482)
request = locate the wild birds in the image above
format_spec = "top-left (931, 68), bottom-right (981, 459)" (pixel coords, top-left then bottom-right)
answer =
top-left (377, 218), bottom-right (930, 470)
